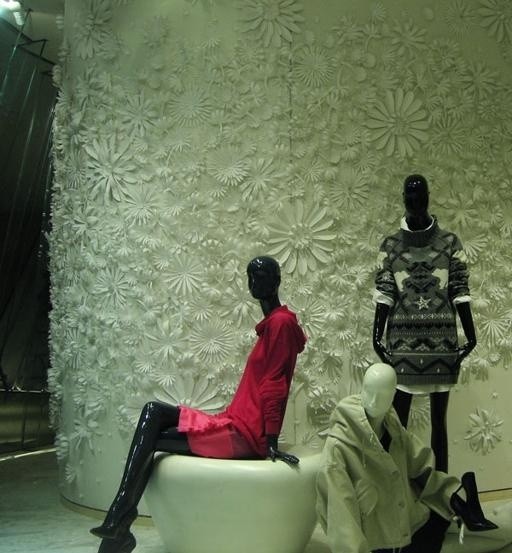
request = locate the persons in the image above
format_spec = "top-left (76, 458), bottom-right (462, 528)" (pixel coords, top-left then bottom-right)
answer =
top-left (370, 172), bottom-right (479, 477)
top-left (301, 361), bottom-right (500, 552)
top-left (88, 255), bottom-right (309, 553)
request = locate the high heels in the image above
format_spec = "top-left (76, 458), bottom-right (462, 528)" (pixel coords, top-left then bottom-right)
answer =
top-left (450, 473), bottom-right (500, 532)
